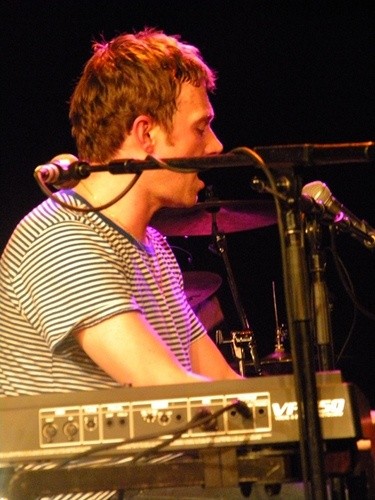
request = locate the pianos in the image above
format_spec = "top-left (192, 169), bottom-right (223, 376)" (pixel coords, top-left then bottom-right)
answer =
top-left (1, 370), bottom-right (356, 462)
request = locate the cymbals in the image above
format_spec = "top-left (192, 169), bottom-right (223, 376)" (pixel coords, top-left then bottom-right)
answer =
top-left (181, 270), bottom-right (223, 308)
top-left (146, 192), bottom-right (280, 236)
top-left (242, 347), bottom-right (293, 367)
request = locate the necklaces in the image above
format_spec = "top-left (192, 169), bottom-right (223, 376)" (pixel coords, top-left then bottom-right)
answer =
top-left (80, 179), bottom-right (144, 248)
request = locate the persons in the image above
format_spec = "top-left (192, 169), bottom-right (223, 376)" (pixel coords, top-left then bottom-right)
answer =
top-left (0, 28), bottom-right (248, 500)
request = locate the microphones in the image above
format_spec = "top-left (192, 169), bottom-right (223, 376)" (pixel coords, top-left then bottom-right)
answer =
top-left (301, 180), bottom-right (375, 251)
top-left (33, 153), bottom-right (80, 190)
